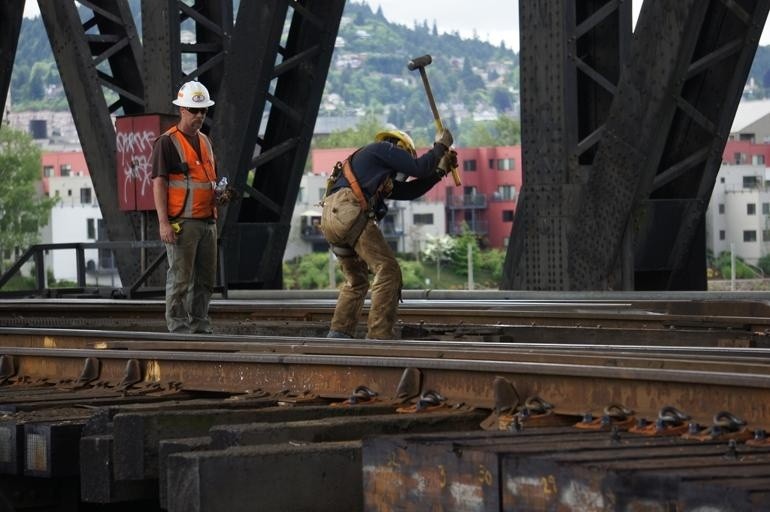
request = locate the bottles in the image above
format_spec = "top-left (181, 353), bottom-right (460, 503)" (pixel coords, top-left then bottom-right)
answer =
top-left (216, 177), bottom-right (228, 200)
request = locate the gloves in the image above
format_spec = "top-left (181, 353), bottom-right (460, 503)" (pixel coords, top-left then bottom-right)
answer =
top-left (435, 126), bottom-right (459, 175)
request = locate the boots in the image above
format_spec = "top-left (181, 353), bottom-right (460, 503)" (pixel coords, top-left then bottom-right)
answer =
top-left (326, 331), bottom-right (354, 340)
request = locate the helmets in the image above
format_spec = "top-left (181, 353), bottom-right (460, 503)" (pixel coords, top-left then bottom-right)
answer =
top-left (374, 130), bottom-right (419, 159)
top-left (172, 81), bottom-right (215, 108)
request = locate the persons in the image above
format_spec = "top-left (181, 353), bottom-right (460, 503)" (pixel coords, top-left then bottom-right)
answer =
top-left (151, 81), bottom-right (230, 334)
top-left (320, 129), bottom-right (459, 340)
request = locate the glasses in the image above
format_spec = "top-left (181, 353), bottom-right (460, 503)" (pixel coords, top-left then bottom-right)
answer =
top-left (184, 107), bottom-right (208, 115)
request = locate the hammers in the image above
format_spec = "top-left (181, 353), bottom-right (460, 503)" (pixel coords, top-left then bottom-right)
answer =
top-left (409, 55), bottom-right (462, 186)
